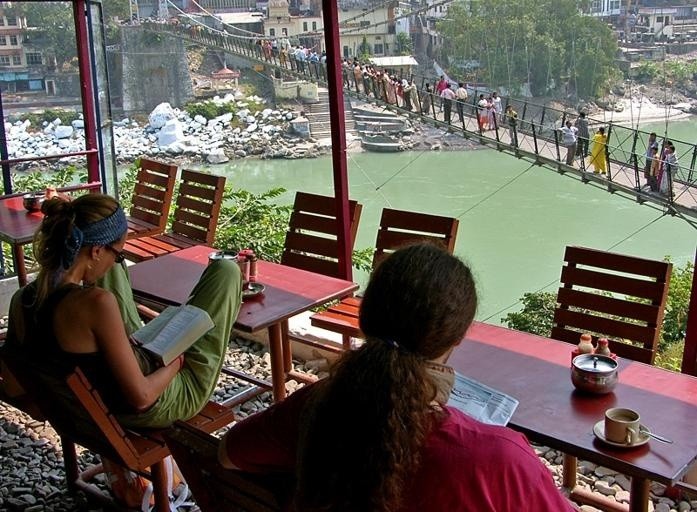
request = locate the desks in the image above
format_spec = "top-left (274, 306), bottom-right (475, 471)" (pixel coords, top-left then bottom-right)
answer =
top-left (443, 321), bottom-right (697, 512)
top-left (125, 245), bottom-right (360, 407)
top-left (0, 192), bottom-right (76, 288)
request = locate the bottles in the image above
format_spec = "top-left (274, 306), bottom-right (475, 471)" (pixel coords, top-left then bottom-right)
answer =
top-left (237, 250), bottom-right (257, 281)
top-left (604, 407), bottom-right (640, 446)
top-left (578, 334), bottom-right (593, 353)
top-left (595, 338), bottom-right (610, 357)
top-left (45, 188), bottom-right (58, 199)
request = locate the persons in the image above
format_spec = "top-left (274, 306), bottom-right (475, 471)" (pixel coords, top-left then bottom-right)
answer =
top-left (456, 82), bottom-right (468, 126)
top-left (440, 85), bottom-right (456, 123)
top-left (247, 38), bottom-right (328, 84)
top-left (558, 120), bottom-right (578, 165)
top-left (476, 91), bottom-right (503, 133)
top-left (339, 58), bottom-right (433, 118)
top-left (216, 236), bottom-right (587, 512)
top-left (2, 191), bottom-right (242, 429)
top-left (644, 133), bottom-right (679, 194)
top-left (588, 126), bottom-right (608, 173)
top-left (504, 106), bottom-right (520, 146)
top-left (120, 16), bottom-right (229, 49)
top-left (436, 77), bottom-right (449, 112)
top-left (574, 112), bottom-right (590, 157)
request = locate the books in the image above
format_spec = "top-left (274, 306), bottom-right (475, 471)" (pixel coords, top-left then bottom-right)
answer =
top-left (126, 296), bottom-right (215, 367)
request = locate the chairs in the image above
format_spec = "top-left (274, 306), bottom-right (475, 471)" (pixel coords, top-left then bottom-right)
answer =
top-left (125, 158), bottom-right (178, 239)
top-left (122, 170), bottom-right (226, 263)
top-left (277, 192), bottom-right (362, 377)
top-left (551, 245), bottom-right (672, 491)
top-left (162, 419), bottom-right (300, 512)
top-left (1, 345), bottom-right (234, 512)
top-left (310, 208), bottom-right (459, 354)
top-left (680, 248), bottom-right (696, 378)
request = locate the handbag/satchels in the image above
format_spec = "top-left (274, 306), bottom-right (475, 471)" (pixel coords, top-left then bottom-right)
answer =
top-left (101, 457), bottom-right (182, 508)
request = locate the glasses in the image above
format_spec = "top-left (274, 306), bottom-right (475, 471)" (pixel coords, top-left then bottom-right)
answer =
top-left (105, 244), bottom-right (127, 263)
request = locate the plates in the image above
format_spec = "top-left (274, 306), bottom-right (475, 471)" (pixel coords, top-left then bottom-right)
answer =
top-left (241, 283), bottom-right (265, 297)
top-left (593, 419), bottom-right (652, 448)
